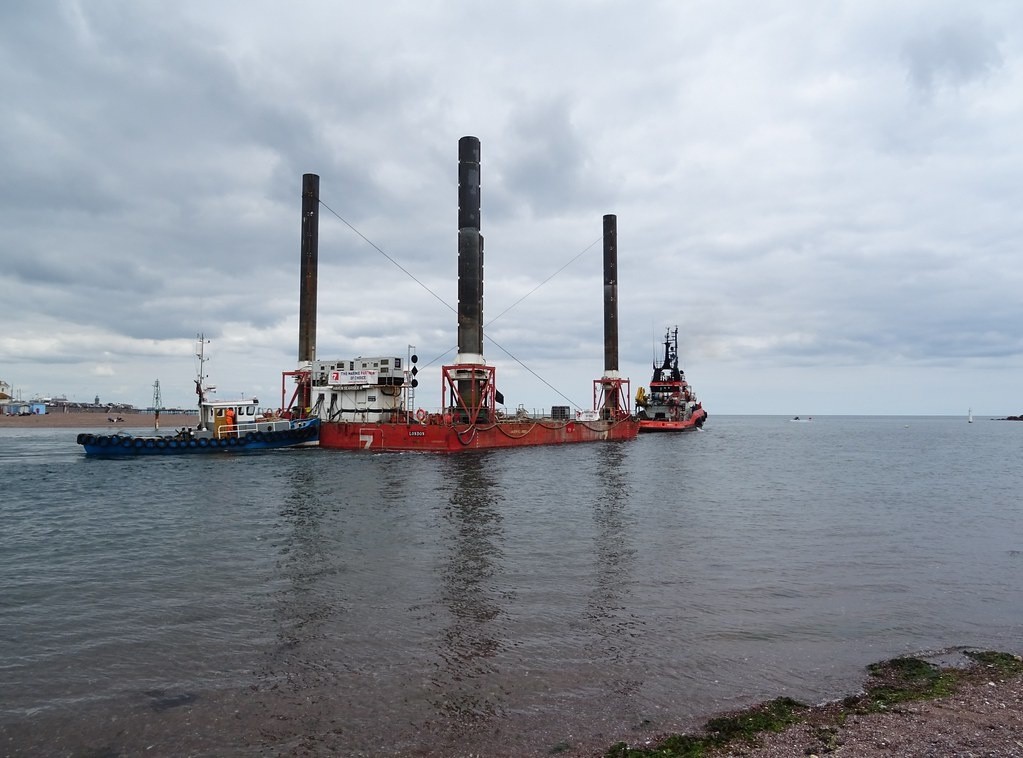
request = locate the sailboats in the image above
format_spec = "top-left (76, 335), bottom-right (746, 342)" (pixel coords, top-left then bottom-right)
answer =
top-left (967, 410), bottom-right (973, 423)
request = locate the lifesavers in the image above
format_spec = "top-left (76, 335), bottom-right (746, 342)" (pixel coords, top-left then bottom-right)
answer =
top-left (669, 408), bottom-right (674, 414)
top-left (702, 415), bottom-right (705, 421)
top-left (696, 418), bottom-right (701, 427)
top-left (265, 424), bottom-right (316, 442)
top-left (705, 412), bottom-right (707, 418)
top-left (76, 430), bottom-right (265, 448)
top-left (417, 409), bottom-right (425, 420)
top-left (686, 391), bottom-right (688, 396)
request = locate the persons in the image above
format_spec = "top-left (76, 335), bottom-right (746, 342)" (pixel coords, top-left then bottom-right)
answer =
top-left (226, 407), bottom-right (234, 431)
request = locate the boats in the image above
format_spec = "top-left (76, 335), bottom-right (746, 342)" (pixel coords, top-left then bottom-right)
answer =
top-left (636, 326), bottom-right (707, 432)
top-left (75, 332), bottom-right (321, 459)
